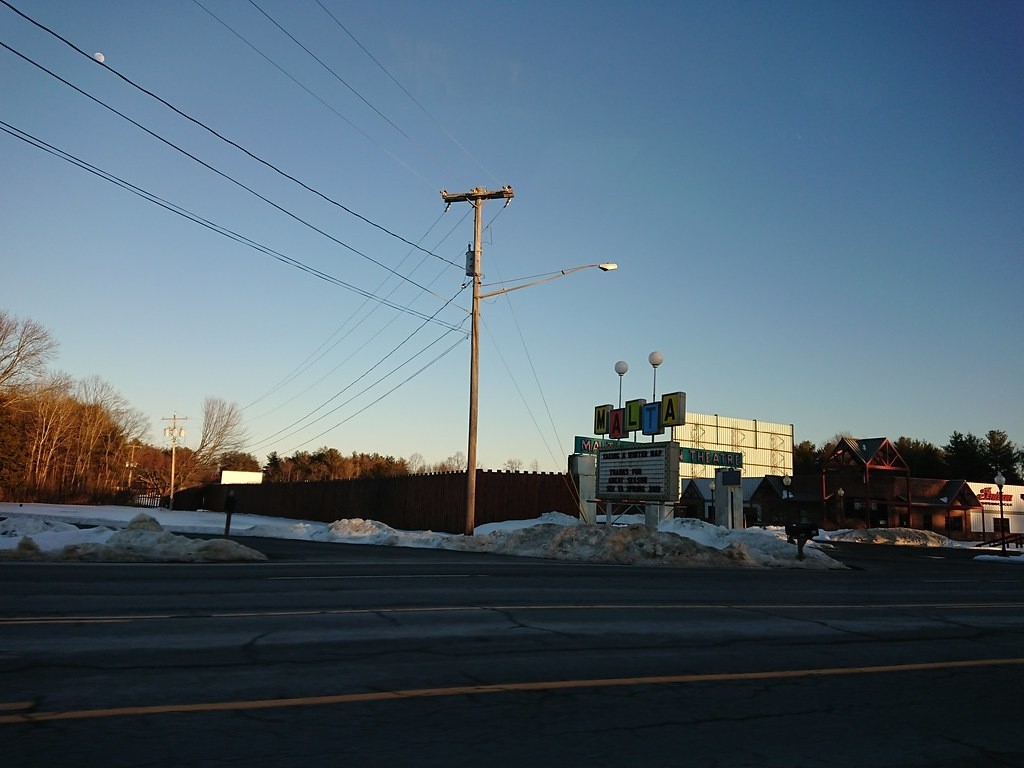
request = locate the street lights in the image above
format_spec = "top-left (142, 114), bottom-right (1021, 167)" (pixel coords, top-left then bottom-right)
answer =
top-left (466, 260), bottom-right (619, 538)
top-left (993, 471), bottom-right (1010, 557)
